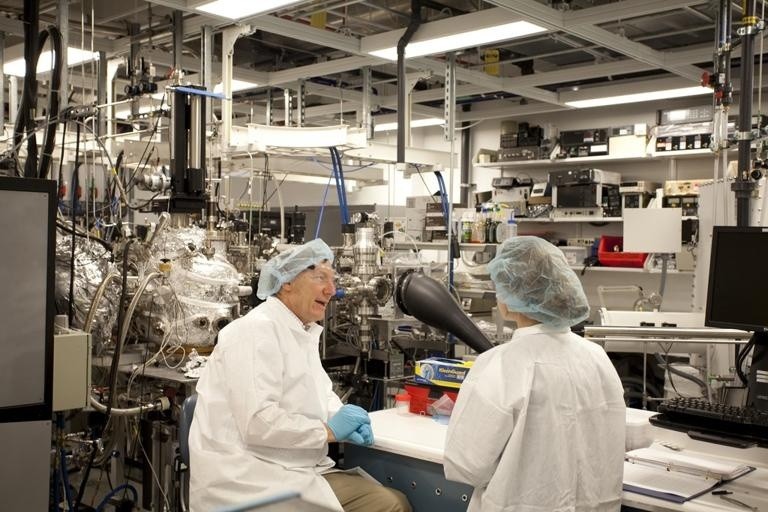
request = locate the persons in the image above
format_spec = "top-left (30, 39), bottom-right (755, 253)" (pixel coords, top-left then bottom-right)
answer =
top-left (187, 238), bottom-right (415, 512)
top-left (441, 236), bottom-right (629, 511)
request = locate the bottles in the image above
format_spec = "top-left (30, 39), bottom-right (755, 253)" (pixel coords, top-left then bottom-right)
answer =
top-left (395, 394), bottom-right (411, 416)
top-left (459, 212), bottom-right (518, 243)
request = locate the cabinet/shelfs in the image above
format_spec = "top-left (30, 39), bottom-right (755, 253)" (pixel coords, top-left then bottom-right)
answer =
top-left (475, 148), bottom-right (714, 276)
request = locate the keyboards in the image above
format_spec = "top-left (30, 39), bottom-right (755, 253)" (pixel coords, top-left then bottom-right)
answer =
top-left (648, 398), bottom-right (767, 449)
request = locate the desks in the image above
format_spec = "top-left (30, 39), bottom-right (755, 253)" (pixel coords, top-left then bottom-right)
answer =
top-left (342, 398), bottom-right (768, 510)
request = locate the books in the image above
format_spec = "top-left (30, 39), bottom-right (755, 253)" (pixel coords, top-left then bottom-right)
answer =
top-left (621, 444), bottom-right (756, 502)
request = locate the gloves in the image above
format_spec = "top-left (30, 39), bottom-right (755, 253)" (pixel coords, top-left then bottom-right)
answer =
top-left (347, 423), bottom-right (374, 446)
top-left (327, 404), bottom-right (370, 440)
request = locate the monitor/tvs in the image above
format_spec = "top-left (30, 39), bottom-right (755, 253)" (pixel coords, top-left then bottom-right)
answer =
top-left (703, 226), bottom-right (767, 407)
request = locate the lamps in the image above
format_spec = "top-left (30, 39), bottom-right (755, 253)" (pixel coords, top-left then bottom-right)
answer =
top-left (362, 10), bottom-right (549, 59)
top-left (558, 71), bottom-right (712, 110)
top-left (2, 34), bottom-right (103, 77)
top-left (620, 205), bottom-right (686, 311)
